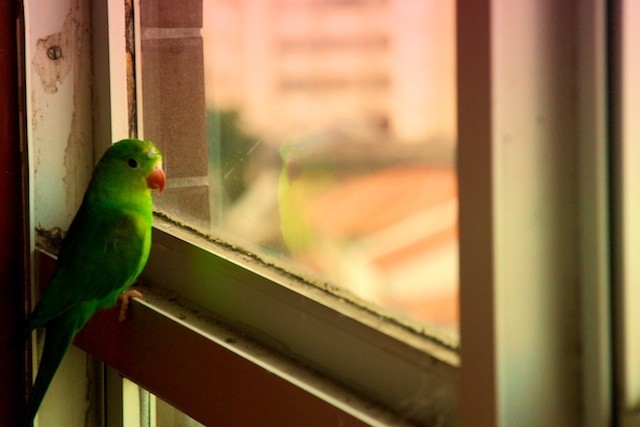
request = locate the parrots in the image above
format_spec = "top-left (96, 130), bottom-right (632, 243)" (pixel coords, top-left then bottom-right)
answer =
top-left (7, 139), bottom-right (167, 427)
